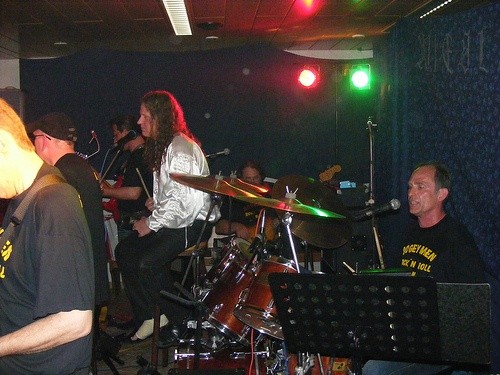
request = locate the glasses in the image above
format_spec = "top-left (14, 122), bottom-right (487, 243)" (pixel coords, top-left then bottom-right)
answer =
top-left (32, 133), bottom-right (51, 141)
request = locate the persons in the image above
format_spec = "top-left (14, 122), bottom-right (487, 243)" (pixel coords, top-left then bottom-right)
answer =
top-left (25, 91), bottom-right (277, 343)
top-left (361, 161), bottom-right (489, 375)
top-left (0, 99), bottom-right (95, 375)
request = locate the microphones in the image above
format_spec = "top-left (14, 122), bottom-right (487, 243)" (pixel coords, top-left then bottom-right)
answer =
top-left (109, 128), bottom-right (138, 153)
top-left (248, 233), bottom-right (263, 253)
top-left (206, 148), bottom-right (231, 158)
top-left (353, 199), bottom-right (400, 221)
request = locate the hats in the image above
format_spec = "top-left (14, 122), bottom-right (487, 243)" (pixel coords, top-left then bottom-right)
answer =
top-left (25, 111), bottom-right (78, 142)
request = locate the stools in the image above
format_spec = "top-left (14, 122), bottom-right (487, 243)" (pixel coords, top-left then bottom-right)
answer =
top-left (150, 241), bottom-right (207, 368)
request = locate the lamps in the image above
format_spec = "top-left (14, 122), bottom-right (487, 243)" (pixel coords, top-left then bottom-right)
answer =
top-left (348, 68), bottom-right (371, 90)
top-left (295, 63), bottom-right (320, 88)
